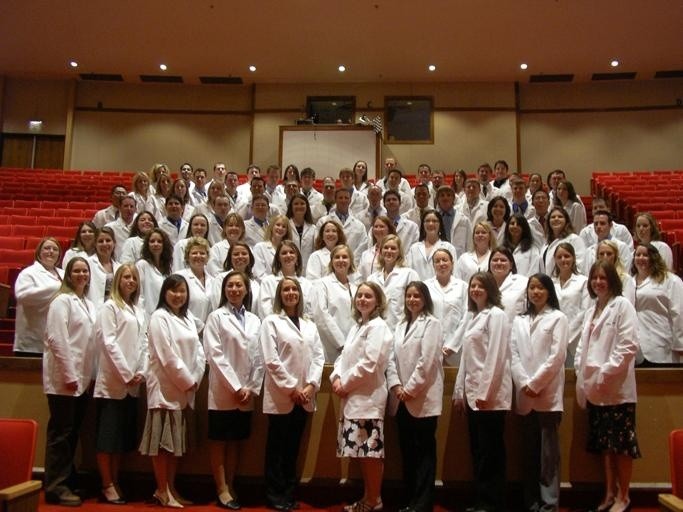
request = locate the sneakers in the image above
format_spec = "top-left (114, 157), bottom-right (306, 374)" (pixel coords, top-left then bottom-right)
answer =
top-left (50, 487), bottom-right (81, 506)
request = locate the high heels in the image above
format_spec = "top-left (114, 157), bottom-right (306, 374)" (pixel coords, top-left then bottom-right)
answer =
top-left (175, 493), bottom-right (193, 505)
top-left (153, 493), bottom-right (184, 509)
top-left (100, 485), bottom-right (126, 504)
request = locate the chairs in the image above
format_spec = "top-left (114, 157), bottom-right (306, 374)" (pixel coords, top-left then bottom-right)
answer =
top-left (401, 173), bottom-right (549, 192)
top-left (46, 224), bottom-right (79, 238)
top-left (672, 178), bottom-right (683, 183)
top-left (31, 207), bottom-right (57, 217)
top-left (672, 189), bottom-right (682, 196)
top-left (613, 172), bottom-right (630, 178)
top-left (0, 214), bottom-right (11, 223)
top-left (53, 239), bottom-right (71, 263)
top-left (626, 178), bottom-right (649, 186)
top-left (8, 266), bottom-right (23, 303)
top-left (9, 214), bottom-right (39, 226)
top-left (56, 208), bottom-right (83, 216)
top-left (97, 201), bottom-right (112, 209)
top-left (12, 224), bottom-right (46, 247)
top-left (616, 197), bottom-right (650, 220)
top-left (0, 416), bottom-right (43, 511)
top-left (676, 229), bottom-right (683, 243)
top-left (633, 171), bottom-right (650, 176)
top-left (612, 191), bottom-right (642, 217)
top-left (38, 216), bottom-right (68, 228)
top-left (676, 197), bottom-right (683, 203)
top-left (591, 172), bottom-right (610, 196)
top-left (633, 203), bottom-right (664, 216)
top-left (70, 201), bottom-right (95, 209)
top-left (0, 248), bottom-right (34, 268)
top-left (1, 167), bottom-right (178, 203)
top-left (635, 184), bottom-right (654, 190)
top-left (647, 178), bottom-right (668, 187)
top-left (1, 265), bottom-right (8, 286)
top-left (648, 209), bottom-right (675, 226)
top-left (41, 199), bottom-right (69, 209)
top-left (86, 210), bottom-right (98, 216)
top-left (653, 170), bottom-right (672, 175)
top-left (674, 243), bottom-right (682, 277)
top-left (658, 428), bottom-right (683, 512)
top-left (235, 173), bottom-right (377, 193)
top-left (0, 224), bottom-right (14, 236)
top-left (674, 170), bottom-right (683, 173)
top-left (0, 236), bottom-right (25, 249)
top-left (601, 179), bottom-right (623, 202)
top-left (642, 175), bottom-right (659, 179)
top-left (594, 174), bottom-right (616, 202)
top-left (0, 207), bottom-right (31, 214)
top-left (25, 237), bottom-right (45, 249)
top-left (646, 190), bottom-right (666, 198)
top-left (608, 185), bottom-right (633, 209)
top-left (657, 184), bottom-right (679, 192)
top-left (619, 175), bottom-right (639, 180)
top-left (667, 230), bottom-right (676, 249)
top-left (14, 199), bottom-right (41, 208)
top-left (668, 203), bottom-right (682, 211)
top-left (676, 210), bottom-right (683, 220)
top-left (67, 218), bottom-right (90, 225)
top-left (654, 197), bottom-right (673, 203)
top-left (660, 219), bottom-right (683, 232)
top-left (661, 175), bottom-right (680, 180)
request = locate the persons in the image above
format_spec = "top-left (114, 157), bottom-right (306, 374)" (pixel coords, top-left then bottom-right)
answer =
top-left (525, 172), bottom-right (545, 211)
top-left (12, 237), bottom-right (66, 358)
top-left (236, 164), bottom-right (270, 204)
top-left (508, 173), bottom-right (522, 188)
top-left (356, 215), bottom-right (400, 287)
top-left (580, 195), bottom-right (634, 248)
top-left (202, 270), bottom-right (266, 510)
top-left (160, 195), bottom-right (190, 246)
top-left (85, 226), bottom-right (123, 316)
top-left (253, 214), bottom-right (291, 282)
top-left (272, 164), bottom-right (301, 204)
top-left (205, 162), bottom-right (227, 196)
top-left (172, 214), bottom-right (210, 272)
top-left (476, 163), bottom-right (503, 204)
top-left (487, 246), bottom-right (529, 329)
top-left (424, 248), bottom-right (469, 367)
top-left (82, 262), bottom-right (141, 506)
top-left (260, 275), bottom-right (325, 512)
top-left (61, 220), bottom-right (97, 270)
top-left (287, 194), bottom-right (317, 277)
top-left (353, 160), bottom-right (373, 197)
top-left (435, 185), bottom-right (473, 261)
top-left (189, 168), bottom-right (210, 217)
top-left (127, 170), bottom-right (160, 224)
top-left (547, 170), bottom-right (582, 211)
top-left (375, 156), bottom-right (410, 194)
top-left (633, 212), bottom-right (673, 273)
top-left (206, 195), bottom-right (231, 246)
top-left (258, 239), bottom-right (317, 321)
top-left (327, 282), bottom-right (387, 512)
top-left (265, 164), bottom-right (281, 195)
top-left (149, 163), bottom-right (168, 196)
top-left (367, 234), bottom-right (420, 335)
top-left (597, 240), bottom-right (636, 309)
top-left (550, 242), bottom-right (593, 368)
top-left (278, 180), bottom-right (300, 216)
top-left (168, 178), bottom-right (194, 223)
top-left (555, 180), bottom-right (587, 235)
top-left (451, 168), bottom-right (467, 213)
top-left (178, 163), bottom-right (195, 192)
top-left (507, 273), bottom-right (569, 512)
top-left (383, 189), bottom-right (421, 259)
top-left (411, 163), bottom-right (434, 196)
top-left (402, 183), bottom-right (436, 231)
top-left (41, 257), bottom-right (97, 507)
top-left (355, 185), bottom-right (387, 235)
top-left (224, 171), bottom-right (249, 221)
top-left (192, 180), bottom-right (225, 217)
top-left (313, 188), bottom-right (368, 270)
top-left (387, 280), bottom-right (445, 512)
top-left (138, 273), bottom-right (206, 508)
top-left (585, 209), bottom-right (633, 276)
top-left (508, 179), bottom-right (536, 220)
top-left (451, 271), bottom-right (512, 512)
top-left (206, 212), bottom-right (253, 278)
top-left (489, 160), bottom-right (513, 199)
top-left (632, 243), bottom-right (683, 367)
top-left (174, 236), bottom-right (221, 342)
top-left (337, 166), bottom-right (371, 235)
top-left (120, 210), bottom-right (159, 267)
top-left (154, 174), bottom-right (173, 217)
top-left (527, 190), bottom-right (552, 250)
top-left (500, 213), bottom-right (540, 279)
top-left (457, 178), bottom-right (489, 252)
top-left (430, 169), bottom-right (445, 198)
top-left (208, 242), bottom-right (261, 318)
top-left (246, 177), bottom-right (279, 218)
top-left (134, 227), bottom-right (173, 317)
top-left (538, 207), bottom-right (587, 277)
top-left (313, 177), bottom-right (339, 222)
top-left (305, 220), bottom-right (349, 281)
top-left (573, 261), bottom-right (640, 512)
top-left (315, 244), bottom-right (363, 363)
top-left (299, 167), bottom-right (327, 224)
top-left (457, 221), bottom-right (496, 283)
top-left (243, 194), bottom-right (272, 246)
top-left (486, 195), bottom-right (511, 250)
top-left (90, 185), bottom-right (128, 230)
top-left (104, 195), bottom-right (140, 263)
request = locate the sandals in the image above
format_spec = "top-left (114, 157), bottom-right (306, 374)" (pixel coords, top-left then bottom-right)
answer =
top-left (344, 500), bottom-right (383, 512)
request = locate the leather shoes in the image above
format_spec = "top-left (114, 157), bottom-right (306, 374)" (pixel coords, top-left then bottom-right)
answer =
top-left (216, 497), bottom-right (240, 511)
top-left (264, 496), bottom-right (289, 511)
top-left (289, 500), bottom-right (299, 510)
